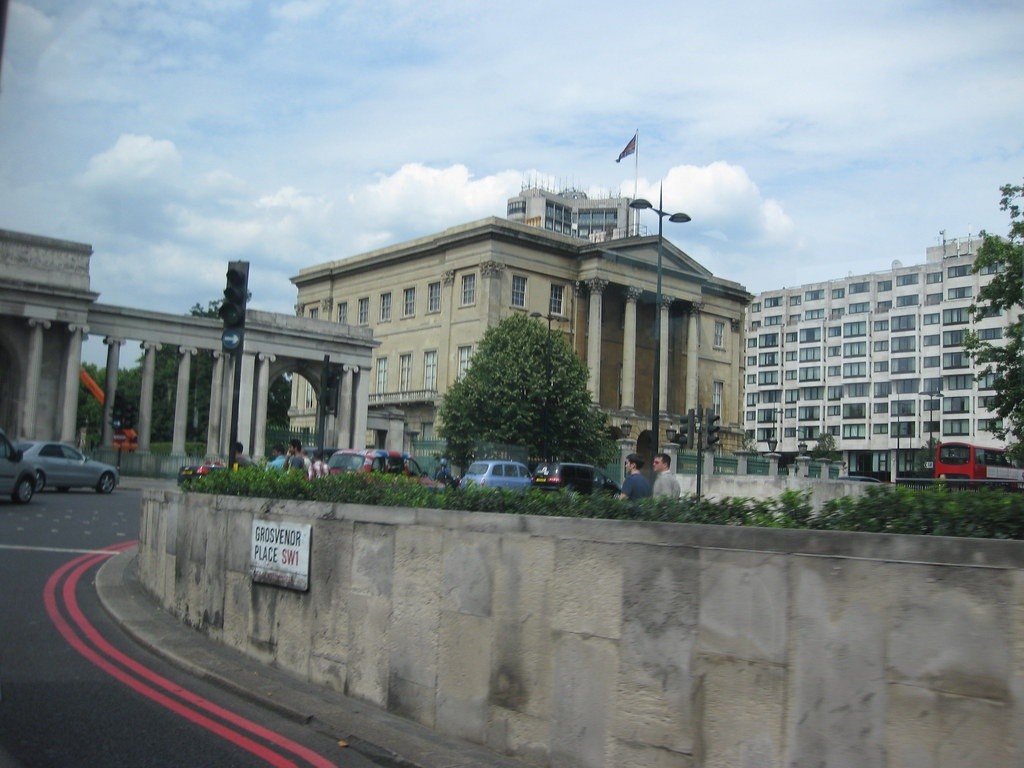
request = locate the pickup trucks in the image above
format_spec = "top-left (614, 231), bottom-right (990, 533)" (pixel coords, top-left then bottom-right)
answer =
top-left (178, 464), bottom-right (225, 486)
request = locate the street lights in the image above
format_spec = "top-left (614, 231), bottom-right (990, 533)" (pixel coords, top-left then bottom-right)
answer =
top-left (773, 403), bottom-right (784, 437)
top-left (919, 392), bottom-right (944, 461)
top-left (629, 179), bottom-right (691, 497)
top-left (530, 300), bottom-right (569, 461)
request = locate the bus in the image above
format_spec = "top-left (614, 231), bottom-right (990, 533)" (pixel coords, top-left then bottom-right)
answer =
top-left (934, 442), bottom-right (1024, 488)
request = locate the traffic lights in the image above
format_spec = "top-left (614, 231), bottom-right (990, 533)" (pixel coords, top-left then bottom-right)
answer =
top-left (706, 408), bottom-right (720, 446)
top-left (125, 405), bottom-right (137, 428)
top-left (680, 409), bottom-right (695, 448)
top-left (109, 404), bottom-right (123, 428)
top-left (219, 259), bottom-right (250, 327)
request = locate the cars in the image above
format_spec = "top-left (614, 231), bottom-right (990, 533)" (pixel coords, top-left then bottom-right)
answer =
top-left (326, 449), bottom-right (445, 489)
top-left (839, 476), bottom-right (881, 482)
top-left (0, 429), bottom-right (34, 504)
top-left (458, 460), bottom-right (532, 495)
top-left (17, 440), bottom-right (120, 495)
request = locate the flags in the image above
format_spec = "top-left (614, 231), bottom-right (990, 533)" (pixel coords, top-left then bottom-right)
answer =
top-left (616, 135), bottom-right (636, 163)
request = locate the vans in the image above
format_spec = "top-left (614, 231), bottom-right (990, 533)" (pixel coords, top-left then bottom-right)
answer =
top-left (531, 462), bottom-right (621, 499)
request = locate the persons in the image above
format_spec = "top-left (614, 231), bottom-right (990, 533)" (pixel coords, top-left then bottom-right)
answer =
top-left (235, 442), bottom-right (252, 469)
top-left (619, 453), bottom-right (652, 501)
top-left (283, 439), bottom-right (304, 469)
top-left (269, 444), bottom-right (286, 469)
top-left (652, 454), bottom-right (680, 499)
top-left (287, 450), bottom-right (330, 481)
top-left (434, 459), bottom-right (448, 480)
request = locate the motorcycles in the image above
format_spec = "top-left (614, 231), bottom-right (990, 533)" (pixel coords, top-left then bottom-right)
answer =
top-left (447, 475), bottom-right (460, 490)
